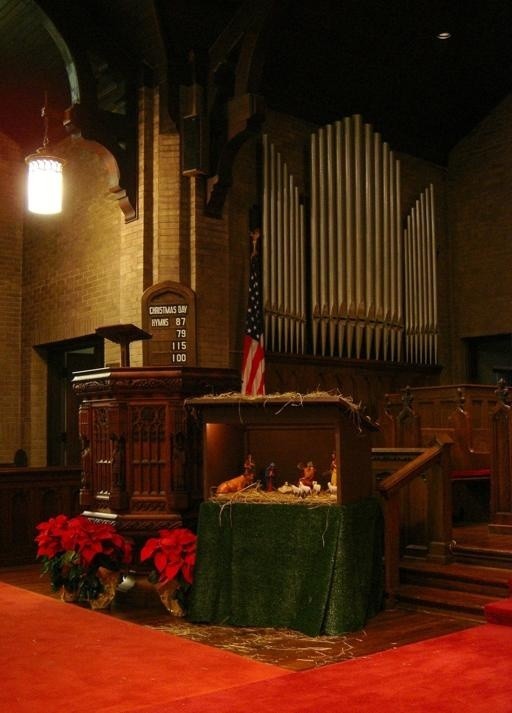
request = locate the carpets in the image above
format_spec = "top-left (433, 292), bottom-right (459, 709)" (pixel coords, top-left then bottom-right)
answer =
top-left (1, 582), bottom-right (512, 713)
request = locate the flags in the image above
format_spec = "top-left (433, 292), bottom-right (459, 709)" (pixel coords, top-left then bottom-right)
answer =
top-left (241, 255), bottom-right (268, 396)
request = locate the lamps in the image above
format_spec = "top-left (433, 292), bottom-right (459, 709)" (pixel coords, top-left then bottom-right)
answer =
top-left (24, 107), bottom-right (69, 215)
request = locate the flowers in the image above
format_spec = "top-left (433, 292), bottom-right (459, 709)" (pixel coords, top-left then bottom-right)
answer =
top-left (33, 514), bottom-right (198, 604)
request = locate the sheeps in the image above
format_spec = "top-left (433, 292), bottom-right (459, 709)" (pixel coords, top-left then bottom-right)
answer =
top-left (288, 478), bottom-right (338, 498)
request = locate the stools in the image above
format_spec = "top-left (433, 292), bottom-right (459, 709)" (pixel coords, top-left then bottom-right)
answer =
top-left (450, 468), bottom-right (492, 527)
